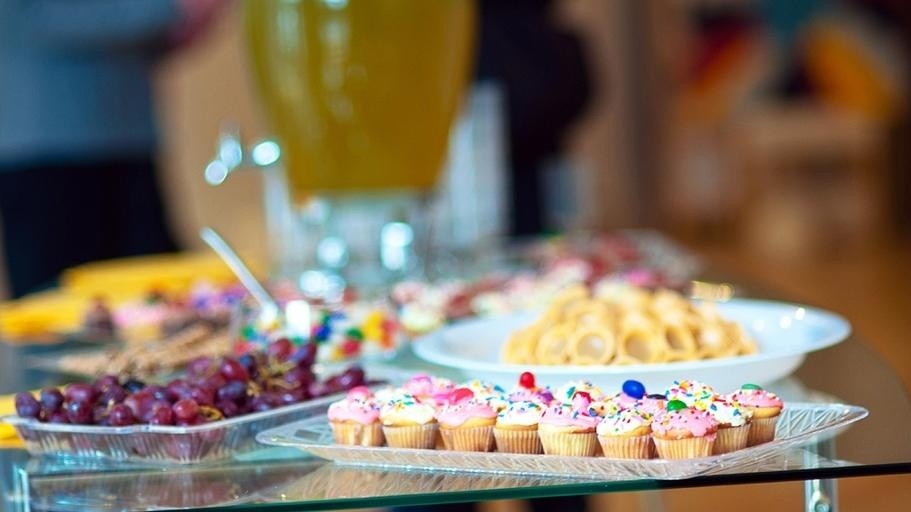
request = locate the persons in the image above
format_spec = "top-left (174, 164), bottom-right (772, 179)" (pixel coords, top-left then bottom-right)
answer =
top-left (0, 0), bottom-right (238, 298)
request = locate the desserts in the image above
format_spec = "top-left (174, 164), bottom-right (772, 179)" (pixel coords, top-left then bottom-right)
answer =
top-left (328, 371), bottom-right (783, 459)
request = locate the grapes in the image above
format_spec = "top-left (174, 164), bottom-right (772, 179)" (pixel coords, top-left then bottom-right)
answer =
top-left (15, 338), bottom-right (386, 460)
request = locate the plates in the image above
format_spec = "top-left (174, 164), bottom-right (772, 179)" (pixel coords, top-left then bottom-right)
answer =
top-left (408, 299), bottom-right (854, 398)
